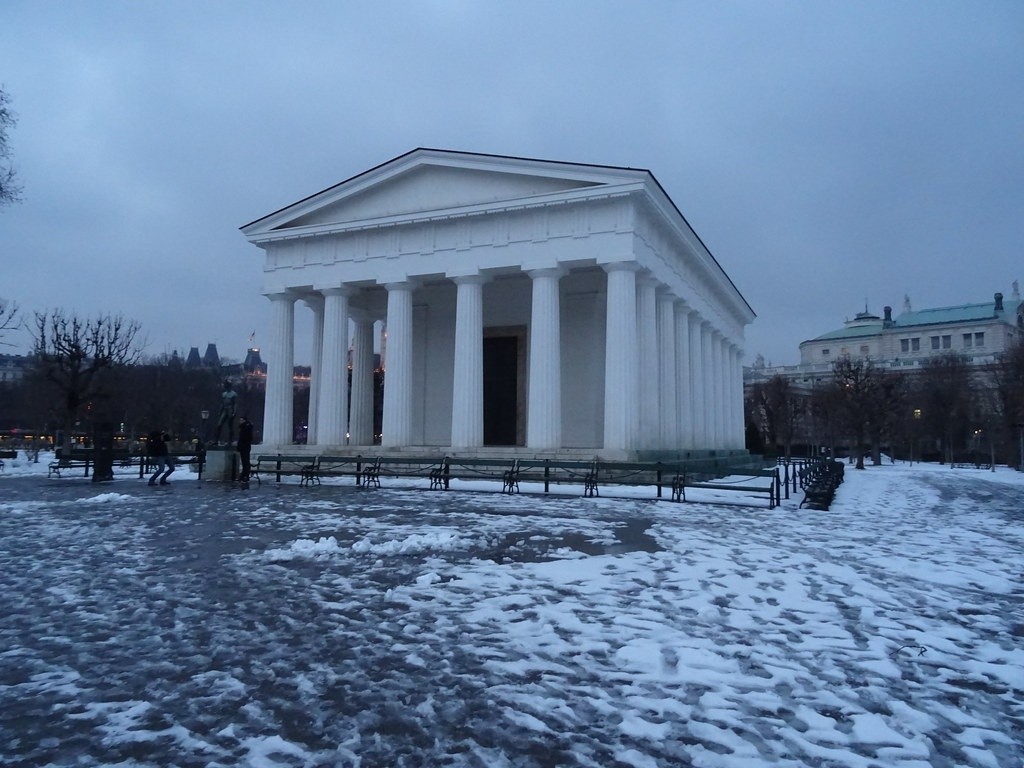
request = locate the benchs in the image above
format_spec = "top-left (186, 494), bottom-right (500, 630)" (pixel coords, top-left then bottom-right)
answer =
top-left (796, 457), bottom-right (845, 511)
top-left (249, 454), bottom-right (776, 511)
top-left (48, 446), bottom-right (204, 479)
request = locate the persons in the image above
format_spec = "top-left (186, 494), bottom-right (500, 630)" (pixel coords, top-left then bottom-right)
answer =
top-left (148, 423), bottom-right (175, 485)
top-left (213, 379), bottom-right (238, 448)
top-left (236, 416), bottom-right (253, 484)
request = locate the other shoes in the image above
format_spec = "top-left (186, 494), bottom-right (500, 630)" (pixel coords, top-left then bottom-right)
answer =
top-left (148, 484), bottom-right (158, 486)
top-left (160, 481), bottom-right (171, 485)
